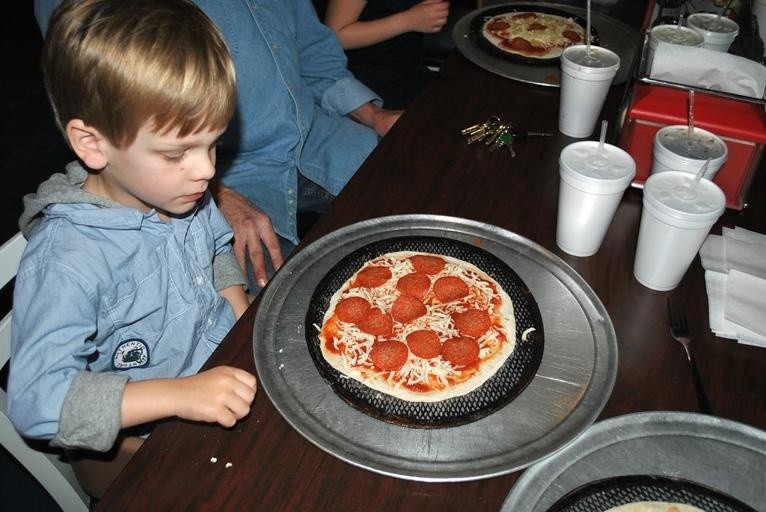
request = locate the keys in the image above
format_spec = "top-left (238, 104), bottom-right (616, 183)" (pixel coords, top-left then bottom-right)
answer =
top-left (460, 114), bottom-right (553, 157)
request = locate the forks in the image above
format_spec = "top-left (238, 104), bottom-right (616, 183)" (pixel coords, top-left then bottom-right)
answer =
top-left (664, 295), bottom-right (715, 415)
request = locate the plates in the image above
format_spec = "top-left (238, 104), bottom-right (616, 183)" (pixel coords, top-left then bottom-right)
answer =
top-left (468, 3), bottom-right (600, 69)
top-left (543, 473), bottom-right (761, 511)
top-left (304, 234), bottom-right (548, 429)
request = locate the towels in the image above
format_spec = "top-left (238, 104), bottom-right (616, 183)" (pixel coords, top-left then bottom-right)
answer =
top-left (687, 213), bottom-right (766, 351)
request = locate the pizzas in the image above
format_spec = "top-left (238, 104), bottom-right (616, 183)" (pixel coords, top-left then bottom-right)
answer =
top-left (483, 12), bottom-right (586, 60)
top-left (320, 251), bottom-right (516, 403)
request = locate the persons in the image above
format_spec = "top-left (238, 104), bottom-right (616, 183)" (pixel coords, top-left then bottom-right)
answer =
top-left (324, 0), bottom-right (450, 101)
top-left (35, 0), bottom-right (408, 290)
top-left (9, 0), bottom-right (257, 454)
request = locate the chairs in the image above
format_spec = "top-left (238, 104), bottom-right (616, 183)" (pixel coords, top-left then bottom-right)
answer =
top-left (0, 219), bottom-right (94, 511)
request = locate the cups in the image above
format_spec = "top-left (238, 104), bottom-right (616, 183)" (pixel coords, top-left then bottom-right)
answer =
top-left (556, 139), bottom-right (637, 259)
top-left (557, 45), bottom-right (623, 138)
top-left (687, 10), bottom-right (743, 52)
top-left (646, 23), bottom-right (706, 79)
top-left (631, 171), bottom-right (729, 292)
top-left (651, 123), bottom-right (732, 185)
